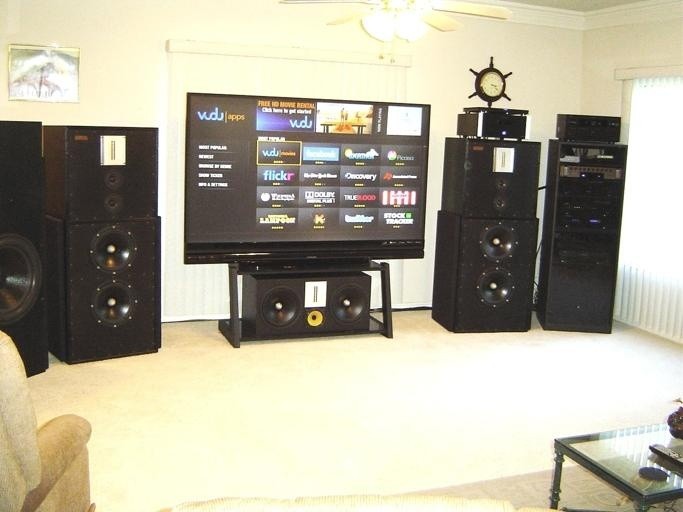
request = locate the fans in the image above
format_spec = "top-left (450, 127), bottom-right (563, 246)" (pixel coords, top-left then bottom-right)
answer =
top-left (275, 0), bottom-right (514, 40)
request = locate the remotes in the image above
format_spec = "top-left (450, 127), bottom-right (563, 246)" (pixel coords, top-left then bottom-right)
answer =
top-left (647, 443), bottom-right (683, 468)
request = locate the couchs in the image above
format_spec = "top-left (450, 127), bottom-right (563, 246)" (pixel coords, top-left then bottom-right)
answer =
top-left (0, 326), bottom-right (96, 511)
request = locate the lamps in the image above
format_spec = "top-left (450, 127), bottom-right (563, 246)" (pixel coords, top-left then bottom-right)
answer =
top-left (356, 4), bottom-right (433, 44)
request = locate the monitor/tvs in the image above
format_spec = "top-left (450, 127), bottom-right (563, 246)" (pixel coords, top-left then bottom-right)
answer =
top-left (184, 88), bottom-right (431, 264)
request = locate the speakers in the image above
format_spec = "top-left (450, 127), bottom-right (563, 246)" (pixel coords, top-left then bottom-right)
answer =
top-left (48, 216), bottom-right (162, 366)
top-left (48, 125), bottom-right (158, 217)
top-left (242, 274), bottom-right (372, 338)
top-left (442, 138), bottom-right (541, 216)
top-left (0, 122), bottom-right (47, 379)
top-left (431, 210), bottom-right (539, 332)
top-left (541, 142), bottom-right (629, 334)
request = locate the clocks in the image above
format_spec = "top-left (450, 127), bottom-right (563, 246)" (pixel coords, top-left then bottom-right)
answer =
top-left (464, 54), bottom-right (513, 111)
